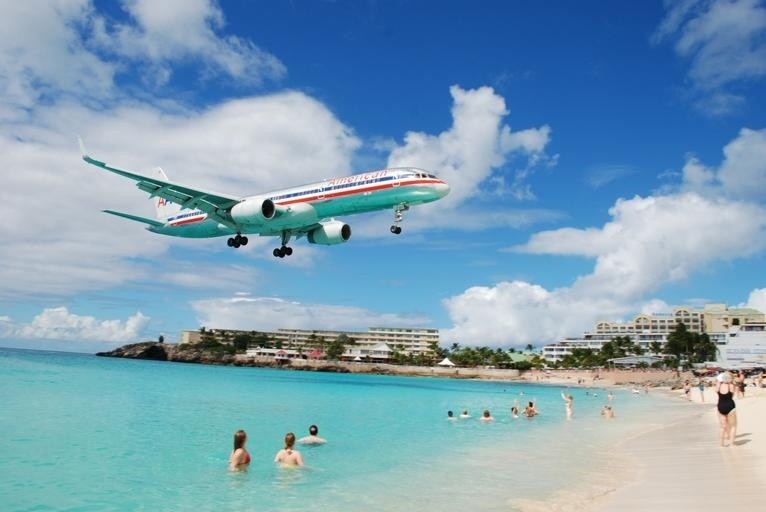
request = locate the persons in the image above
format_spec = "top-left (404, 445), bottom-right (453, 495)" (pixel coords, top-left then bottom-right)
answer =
top-left (228, 429), bottom-right (250, 472)
top-left (275, 433), bottom-right (326, 472)
top-left (448, 366), bottom-right (653, 425)
top-left (683, 370), bottom-right (766, 448)
top-left (297, 425), bottom-right (327, 444)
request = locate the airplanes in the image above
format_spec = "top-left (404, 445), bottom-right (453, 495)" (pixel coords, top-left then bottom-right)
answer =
top-left (76, 134), bottom-right (450, 259)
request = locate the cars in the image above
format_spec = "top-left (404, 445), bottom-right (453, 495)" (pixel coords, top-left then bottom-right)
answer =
top-left (693, 363), bottom-right (766, 377)
